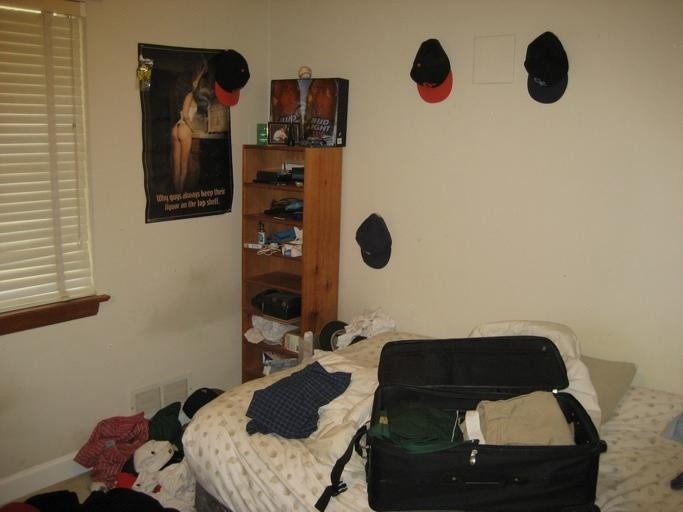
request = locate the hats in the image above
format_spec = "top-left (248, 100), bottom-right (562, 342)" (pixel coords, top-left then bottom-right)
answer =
top-left (356, 213), bottom-right (391, 268)
top-left (410, 38), bottom-right (453, 102)
top-left (524, 31), bottom-right (569, 103)
top-left (214, 49), bottom-right (249, 107)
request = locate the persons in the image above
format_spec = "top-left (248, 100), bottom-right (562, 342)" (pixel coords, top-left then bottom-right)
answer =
top-left (171, 72), bottom-right (203, 192)
top-left (273, 126), bottom-right (288, 142)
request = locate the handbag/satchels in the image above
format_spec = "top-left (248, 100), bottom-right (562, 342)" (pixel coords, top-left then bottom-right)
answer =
top-left (252, 288), bottom-right (302, 320)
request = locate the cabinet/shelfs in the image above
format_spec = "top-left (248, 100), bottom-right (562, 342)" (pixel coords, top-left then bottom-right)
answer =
top-left (241, 144), bottom-right (343, 385)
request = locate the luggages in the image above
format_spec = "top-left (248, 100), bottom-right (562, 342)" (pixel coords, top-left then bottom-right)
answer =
top-left (355, 336), bottom-right (608, 511)
